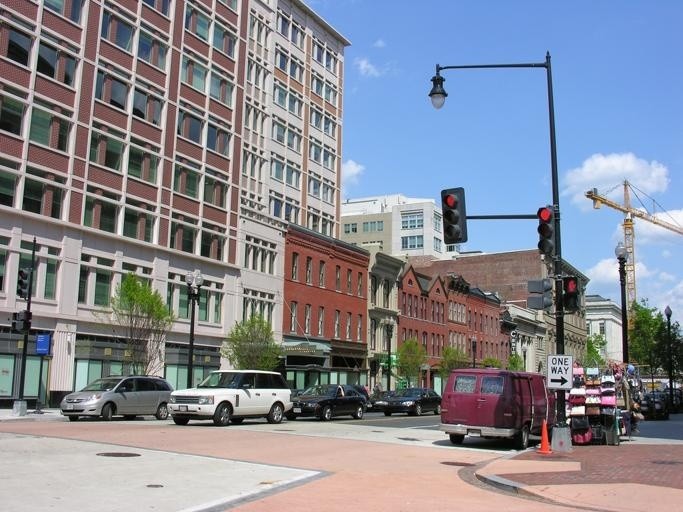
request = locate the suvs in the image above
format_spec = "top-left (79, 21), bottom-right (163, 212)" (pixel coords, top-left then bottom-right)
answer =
top-left (348, 385), bottom-right (369, 400)
top-left (167, 369), bottom-right (294, 425)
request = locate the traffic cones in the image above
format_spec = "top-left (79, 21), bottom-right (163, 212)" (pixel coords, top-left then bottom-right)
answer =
top-left (535, 418), bottom-right (553, 455)
top-left (664, 305), bottom-right (674, 408)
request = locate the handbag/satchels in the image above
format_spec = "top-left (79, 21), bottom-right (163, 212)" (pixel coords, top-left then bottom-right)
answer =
top-left (554, 365), bottom-right (644, 445)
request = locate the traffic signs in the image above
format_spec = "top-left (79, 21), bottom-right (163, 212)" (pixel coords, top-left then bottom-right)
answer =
top-left (546, 354), bottom-right (573, 389)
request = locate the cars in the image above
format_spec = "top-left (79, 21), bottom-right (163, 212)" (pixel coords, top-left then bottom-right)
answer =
top-left (375, 388), bottom-right (442, 416)
top-left (636, 386), bottom-right (683, 420)
top-left (366, 391), bottom-right (395, 413)
top-left (283, 384), bottom-right (367, 421)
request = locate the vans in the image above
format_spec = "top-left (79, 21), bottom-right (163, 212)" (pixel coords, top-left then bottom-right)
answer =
top-left (439, 368), bottom-right (555, 450)
top-left (59, 374), bottom-right (175, 420)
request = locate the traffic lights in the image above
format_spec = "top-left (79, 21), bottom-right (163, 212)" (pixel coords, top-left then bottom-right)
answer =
top-left (562, 276), bottom-right (578, 312)
top-left (17, 269), bottom-right (28, 298)
top-left (536, 206), bottom-right (554, 254)
top-left (12, 310), bottom-right (31, 330)
top-left (440, 187), bottom-right (467, 244)
top-left (527, 279), bottom-right (552, 310)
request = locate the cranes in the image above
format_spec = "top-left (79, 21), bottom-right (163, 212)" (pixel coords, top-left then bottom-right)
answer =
top-left (584, 179), bottom-right (683, 342)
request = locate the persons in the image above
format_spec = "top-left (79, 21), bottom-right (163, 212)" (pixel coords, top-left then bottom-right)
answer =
top-left (365, 385), bottom-right (370, 397)
top-left (373, 384), bottom-right (379, 395)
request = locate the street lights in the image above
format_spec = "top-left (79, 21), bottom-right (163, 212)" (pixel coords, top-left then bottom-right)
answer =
top-left (521, 345), bottom-right (528, 371)
top-left (184, 269), bottom-right (203, 388)
top-left (380, 315), bottom-right (394, 390)
top-left (428, 51), bottom-right (574, 455)
top-left (612, 241), bottom-right (630, 364)
top-left (470, 336), bottom-right (477, 368)
